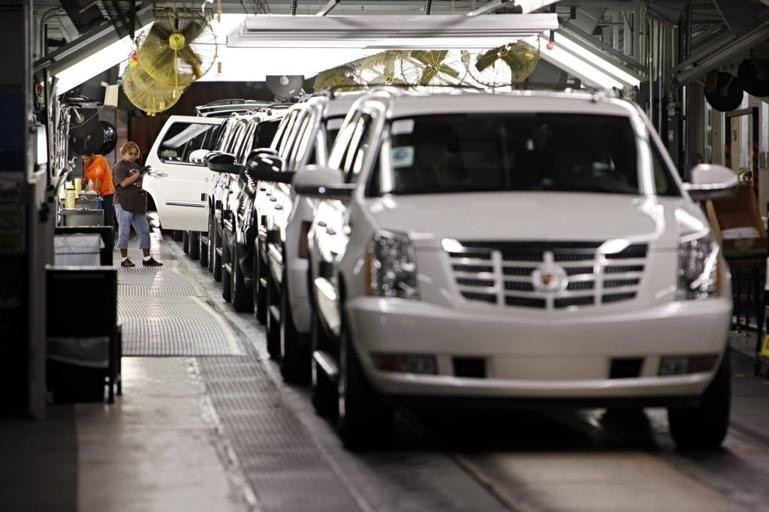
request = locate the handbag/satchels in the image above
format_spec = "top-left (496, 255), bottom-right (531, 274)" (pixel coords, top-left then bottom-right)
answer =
top-left (118, 190), bottom-right (148, 215)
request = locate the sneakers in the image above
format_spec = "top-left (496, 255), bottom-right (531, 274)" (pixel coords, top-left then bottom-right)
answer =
top-left (143, 256), bottom-right (163, 266)
top-left (121, 258), bottom-right (135, 267)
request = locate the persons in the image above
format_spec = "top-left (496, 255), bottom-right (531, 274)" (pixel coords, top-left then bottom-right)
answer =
top-left (80, 146), bottom-right (117, 264)
top-left (112, 140), bottom-right (164, 268)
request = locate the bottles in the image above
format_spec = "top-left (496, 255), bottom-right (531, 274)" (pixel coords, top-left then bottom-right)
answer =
top-left (65, 188), bottom-right (76, 208)
top-left (75, 178), bottom-right (81, 192)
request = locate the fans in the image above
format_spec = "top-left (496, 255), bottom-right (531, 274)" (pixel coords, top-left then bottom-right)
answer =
top-left (312, 35), bottom-right (540, 90)
top-left (122, 7), bottom-right (217, 117)
